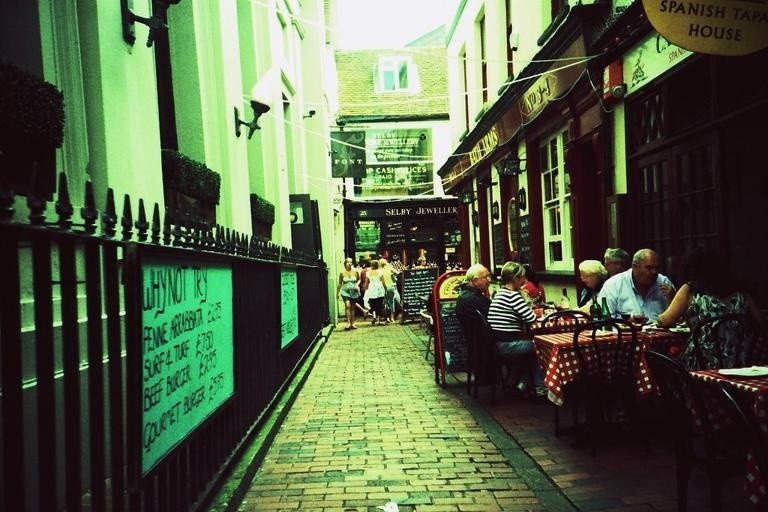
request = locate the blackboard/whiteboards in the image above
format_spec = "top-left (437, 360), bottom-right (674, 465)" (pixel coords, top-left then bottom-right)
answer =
top-left (402, 267), bottom-right (440, 320)
top-left (433, 298), bottom-right (490, 373)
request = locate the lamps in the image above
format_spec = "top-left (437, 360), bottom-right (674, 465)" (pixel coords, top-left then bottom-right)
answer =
top-left (478, 176), bottom-right (498, 188)
top-left (120, 0), bottom-right (182, 48)
top-left (234, 63), bottom-right (281, 140)
top-left (472, 210), bottom-right (478, 227)
top-left (461, 191), bottom-right (479, 204)
top-left (498, 151), bottom-right (528, 177)
top-left (517, 187), bottom-right (526, 211)
top-left (303, 106), bottom-right (316, 120)
top-left (491, 200), bottom-right (499, 221)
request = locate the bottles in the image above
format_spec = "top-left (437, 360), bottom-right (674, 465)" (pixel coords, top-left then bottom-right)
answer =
top-left (601, 296), bottom-right (613, 332)
top-left (445, 261), bottom-right (463, 270)
top-left (491, 286), bottom-right (497, 299)
top-left (561, 289), bottom-right (570, 310)
top-left (589, 293), bottom-right (602, 331)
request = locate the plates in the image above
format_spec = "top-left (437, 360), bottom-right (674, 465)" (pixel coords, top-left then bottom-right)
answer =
top-left (584, 331), bottom-right (613, 338)
top-left (718, 366), bottom-right (768, 378)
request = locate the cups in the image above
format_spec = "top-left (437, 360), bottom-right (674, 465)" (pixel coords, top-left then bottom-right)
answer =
top-left (534, 309), bottom-right (544, 318)
top-left (620, 309), bottom-right (631, 320)
top-left (633, 311), bottom-right (645, 324)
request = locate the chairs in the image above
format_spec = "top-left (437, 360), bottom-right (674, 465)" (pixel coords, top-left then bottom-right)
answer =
top-left (644, 311), bottom-right (767, 512)
top-left (417, 296), bottom-right (592, 408)
top-left (534, 311), bottom-right (690, 445)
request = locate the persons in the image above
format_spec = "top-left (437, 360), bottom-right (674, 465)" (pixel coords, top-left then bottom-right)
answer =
top-left (457, 264), bottom-right (499, 366)
top-left (656, 229), bottom-right (765, 399)
top-left (604, 247), bottom-right (631, 278)
top-left (597, 249), bottom-right (678, 325)
top-left (576, 260), bottom-right (609, 310)
top-left (521, 264), bottom-right (549, 301)
top-left (488, 259), bottom-right (555, 399)
top-left (336, 248), bottom-right (435, 332)
